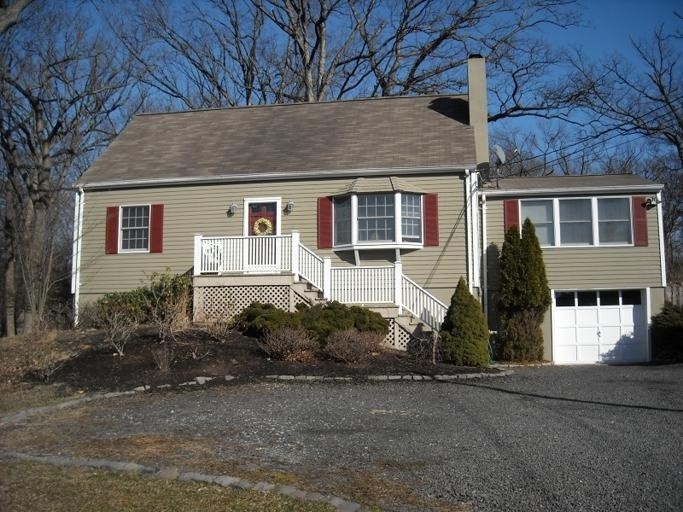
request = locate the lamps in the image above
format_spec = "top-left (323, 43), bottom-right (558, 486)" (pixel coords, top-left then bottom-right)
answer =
top-left (287, 202), bottom-right (295, 212)
top-left (230, 202), bottom-right (238, 214)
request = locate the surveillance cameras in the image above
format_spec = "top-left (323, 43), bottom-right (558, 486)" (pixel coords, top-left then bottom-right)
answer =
top-left (650, 198), bottom-right (657, 206)
top-left (464, 169), bottom-right (470, 176)
